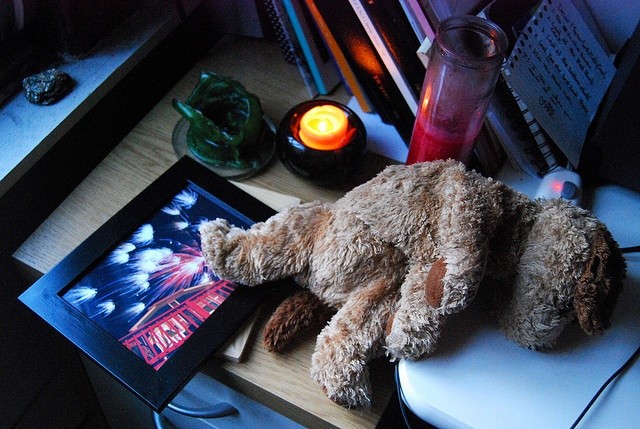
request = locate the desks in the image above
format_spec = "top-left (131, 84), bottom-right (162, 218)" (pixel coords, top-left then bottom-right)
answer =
top-left (0, 0), bottom-right (205, 198)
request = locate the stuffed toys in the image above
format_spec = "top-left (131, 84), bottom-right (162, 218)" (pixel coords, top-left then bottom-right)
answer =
top-left (198, 158), bottom-right (628, 411)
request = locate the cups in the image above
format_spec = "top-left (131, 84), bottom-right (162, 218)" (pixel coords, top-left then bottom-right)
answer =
top-left (405, 13), bottom-right (508, 166)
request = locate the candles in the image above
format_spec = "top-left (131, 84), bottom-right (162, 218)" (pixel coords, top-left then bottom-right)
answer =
top-left (298, 104), bottom-right (348, 150)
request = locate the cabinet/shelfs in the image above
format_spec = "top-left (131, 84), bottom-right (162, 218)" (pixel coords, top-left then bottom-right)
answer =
top-left (12, 33), bottom-right (398, 428)
